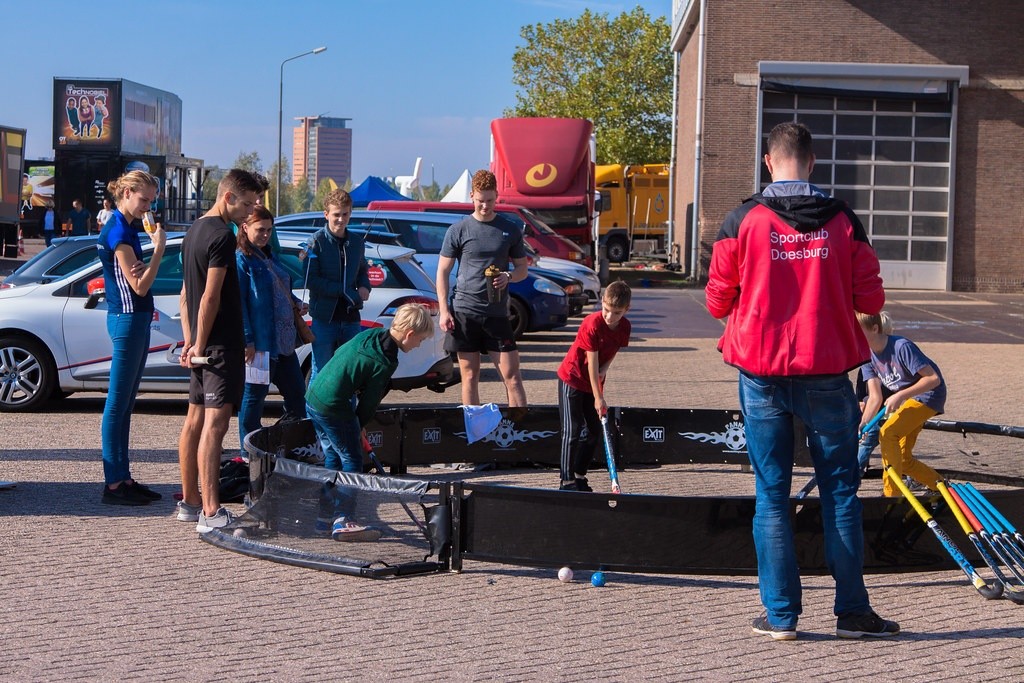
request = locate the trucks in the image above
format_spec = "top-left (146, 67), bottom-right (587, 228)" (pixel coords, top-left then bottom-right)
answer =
top-left (594, 161), bottom-right (671, 264)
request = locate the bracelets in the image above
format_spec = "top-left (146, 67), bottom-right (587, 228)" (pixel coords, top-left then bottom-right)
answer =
top-left (505, 272), bottom-right (513, 283)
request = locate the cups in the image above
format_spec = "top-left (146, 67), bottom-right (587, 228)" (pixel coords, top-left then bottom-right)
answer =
top-left (484, 265), bottom-right (501, 304)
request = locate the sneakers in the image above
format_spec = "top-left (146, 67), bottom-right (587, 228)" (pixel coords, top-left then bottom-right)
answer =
top-left (332, 517), bottom-right (384, 543)
top-left (924, 478), bottom-right (952, 516)
top-left (196, 505), bottom-right (239, 534)
top-left (101, 482), bottom-right (151, 506)
top-left (576, 478), bottom-right (593, 492)
top-left (313, 517), bottom-right (332, 539)
top-left (837, 608), bottom-right (900, 639)
top-left (879, 494), bottom-right (904, 517)
top-left (177, 499), bottom-right (202, 521)
top-left (752, 610), bottom-right (797, 640)
top-left (559, 479), bottom-right (577, 491)
top-left (132, 482), bottom-right (162, 500)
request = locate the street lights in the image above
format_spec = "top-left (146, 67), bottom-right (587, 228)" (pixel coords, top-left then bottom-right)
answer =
top-left (274, 45), bottom-right (329, 218)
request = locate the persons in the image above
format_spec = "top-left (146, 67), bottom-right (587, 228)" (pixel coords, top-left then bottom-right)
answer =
top-left (178, 170), bottom-right (266, 537)
top-left (299, 189), bottom-right (372, 390)
top-left (557, 279), bottom-right (633, 490)
top-left (434, 170), bottom-right (530, 408)
top-left (855, 363), bottom-right (921, 493)
top-left (237, 203), bottom-right (308, 464)
top-left (303, 303), bottom-right (434, 473)
top-left (71, 201), bottom-right (91, 236)
top-left (857, 310), bottom-right (954, 508)
top-left (705, 124), bottom-right (904, 639)
top-left (98, 171), bottom-right (167, 507)
top-left (96, 198), bottom-right (116, 235)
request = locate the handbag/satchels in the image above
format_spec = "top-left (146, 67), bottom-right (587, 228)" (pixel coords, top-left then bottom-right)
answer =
top-left (293, 307), bottom-right (314, 348)
top-left (218, 460), bottom-right (250, 505)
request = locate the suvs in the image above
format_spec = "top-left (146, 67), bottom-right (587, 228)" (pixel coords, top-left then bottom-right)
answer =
top-left (275, 198), bottom-right (602, 342)
top-left (0, 231), bottom-right (455, 416)
top-left (4, 232), bottom-right (187, 287)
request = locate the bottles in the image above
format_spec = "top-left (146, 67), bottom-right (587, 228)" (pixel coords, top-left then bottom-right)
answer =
top-left (140, 211), bottom-right (157, 237)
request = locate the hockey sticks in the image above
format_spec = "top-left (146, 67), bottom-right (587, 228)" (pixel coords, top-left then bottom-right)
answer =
top-left (935, 477), bottom-right (1024, 607)
top-left (796, 406), bottom-right (887, 498)
top-left (598, 374), bottom-right (622, 495)
top-left (165, 340), bottom-right (215, 365)
top-left (883, 462), bottom-right (1005, 600)
top-left (360, 433), bottom-right (429, 540)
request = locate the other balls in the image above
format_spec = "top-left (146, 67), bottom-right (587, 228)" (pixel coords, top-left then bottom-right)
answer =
top-left (591, 571), bottom-right (606, 587)
top-left (558, 566), bottom-right (574, 582)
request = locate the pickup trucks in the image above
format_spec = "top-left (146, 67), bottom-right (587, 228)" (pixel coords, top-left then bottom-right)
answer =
top-left (487, 117), bottom-right (613, 272)
